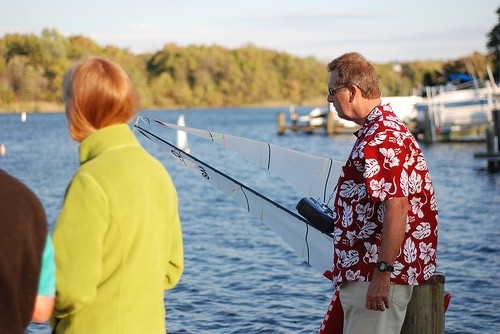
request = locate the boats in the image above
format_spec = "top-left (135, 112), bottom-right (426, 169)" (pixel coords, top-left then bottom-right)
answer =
top-left (298, 65), bottom-right (500, 140)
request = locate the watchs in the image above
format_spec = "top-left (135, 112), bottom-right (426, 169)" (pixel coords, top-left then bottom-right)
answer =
top-left (376, 261), bottom-right (394, 273)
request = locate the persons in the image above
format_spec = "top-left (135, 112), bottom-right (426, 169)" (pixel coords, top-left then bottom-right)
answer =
top-left (45, 56), bottom-right (184, 334)
top-left (0, 169), bottom-right (56, 334)
top-left (319, 53), bottom-right (439, 333)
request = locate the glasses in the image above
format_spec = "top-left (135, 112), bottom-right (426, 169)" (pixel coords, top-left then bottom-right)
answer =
top-left (328, 86), bottom-right (347, 95)
